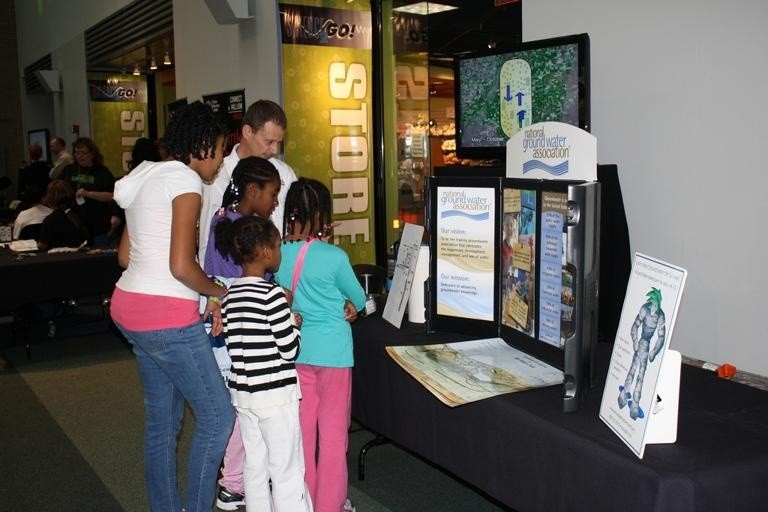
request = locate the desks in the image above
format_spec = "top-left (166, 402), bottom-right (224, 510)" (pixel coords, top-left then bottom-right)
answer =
top-left (318, 309), bottom-right (762, 509)
top-left (0, 233), bottom-right (139, 367)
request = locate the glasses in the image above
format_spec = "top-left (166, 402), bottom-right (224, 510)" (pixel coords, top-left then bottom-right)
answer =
top-left (73, 150), bottom-right (90, 156)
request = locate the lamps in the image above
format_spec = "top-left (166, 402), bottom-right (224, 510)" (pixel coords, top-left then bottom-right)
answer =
top-left (394, 3), bottom-right (463, 18)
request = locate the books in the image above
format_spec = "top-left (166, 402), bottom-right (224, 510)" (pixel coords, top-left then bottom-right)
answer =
top-left (384, 336), bottom-right (565, 408)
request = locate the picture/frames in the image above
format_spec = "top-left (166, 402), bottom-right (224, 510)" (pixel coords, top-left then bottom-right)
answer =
top-left (27, 130), bottom-right (55, 165)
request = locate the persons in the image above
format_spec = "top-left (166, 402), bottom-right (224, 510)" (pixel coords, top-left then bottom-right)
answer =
top-left (274, 177), bottom-right (366, 511)
top-left (191, 98), bottom-right (299, 272)
top-left (213, 215), bottom-right (313, 512)
top-left (109, 99), bottom-right (236, 511)
top-left (38, 177), bottom-right (76, 250)
top-left (49, 138), bottom-right (73, 178)
top-left (18, 144), bottom-right (54, 207)
top-left (59, 137), bottom-right (117, 244)
top-left (195, 154), bottom-right (283, 511)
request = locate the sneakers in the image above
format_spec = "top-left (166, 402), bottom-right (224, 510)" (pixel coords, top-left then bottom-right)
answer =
top-left (216, 488), bottom-right (246, 510)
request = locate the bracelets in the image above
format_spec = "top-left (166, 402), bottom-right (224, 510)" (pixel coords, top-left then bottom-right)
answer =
top-left (207, 279), bottom-right (228, 302)
